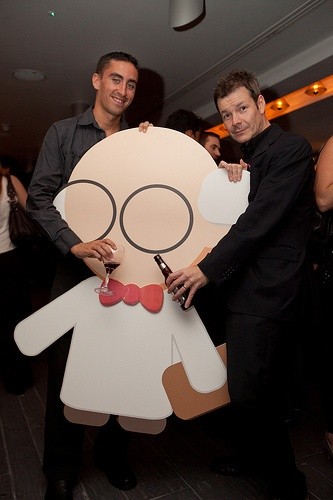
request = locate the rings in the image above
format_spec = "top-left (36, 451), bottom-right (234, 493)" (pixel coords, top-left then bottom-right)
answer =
top-left (183, 285), bottom-right (189, 290)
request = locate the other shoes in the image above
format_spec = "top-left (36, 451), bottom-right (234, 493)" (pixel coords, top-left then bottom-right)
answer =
top-left (2, 355), bottom-right (34, 395)
top-left (324, 431), bottom-right (333, 457)
top-left (275, 470), bottom-right (308, 500)
top-left (209, 457), bottom-right (249, 476)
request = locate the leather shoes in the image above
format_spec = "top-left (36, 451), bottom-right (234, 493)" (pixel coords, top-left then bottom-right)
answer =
top-left (102, 447), bottom-right (136, 490)
top-left (44, 474), bottom-right (73, 500)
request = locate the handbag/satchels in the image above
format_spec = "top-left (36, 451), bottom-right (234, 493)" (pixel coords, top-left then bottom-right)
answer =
top-left (9, 206), bottom-right (40, 249)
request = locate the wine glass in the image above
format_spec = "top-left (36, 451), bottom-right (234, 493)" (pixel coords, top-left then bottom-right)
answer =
top-left (94, 245), bottom-right (123, 296)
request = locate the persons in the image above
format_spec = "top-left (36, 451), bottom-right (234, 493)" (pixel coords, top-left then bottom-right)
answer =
top-left (314, 135), bottom-right (333, 213)
top-left (198, 132), bottom-right (221, 160)
top-left (165, 109), bottom-right (201, 143)
top-left (0, 166), bottom-right (27, 395)
top-left (25, 51), bottom-right (154, 500)
top-left (165, 70), bottom-right (313, 500)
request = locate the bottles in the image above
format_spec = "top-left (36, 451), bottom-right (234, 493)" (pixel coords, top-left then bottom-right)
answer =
top-left (154, 254), bottom-right (195, 312)
